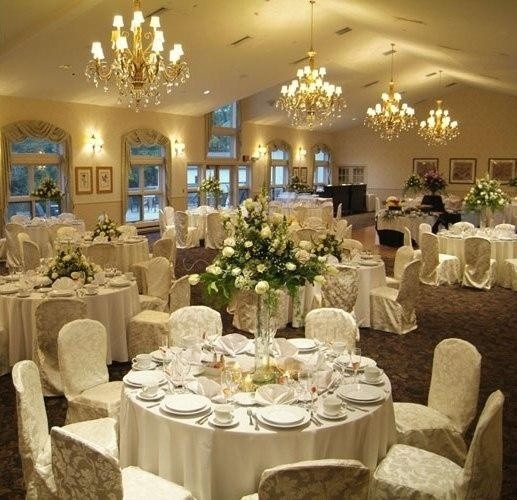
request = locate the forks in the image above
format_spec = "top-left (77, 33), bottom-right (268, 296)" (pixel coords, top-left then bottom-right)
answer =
top-left (247, 410), bottom-right (254, 426)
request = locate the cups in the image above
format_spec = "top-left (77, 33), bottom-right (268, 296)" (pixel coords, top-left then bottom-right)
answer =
top-left (132, 354), bottom-right (153, 368)
top-left (83, 283), bottom-right (98, 292)
top-left (364, 368), bottom-right (383, 381)
top-left (322, 399), bottom-right (346, 415)
top-left (125, 272), bottom-right (133, 281)
top-left (215, 405), bottom-right (234, 423)
top-left (141, 383), bottom-right (158, 397)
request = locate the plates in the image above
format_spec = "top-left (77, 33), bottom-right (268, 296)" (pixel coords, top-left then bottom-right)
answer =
top-left (256, 385), bottom-right (299, 404)
top-left (318, 410), bottom-right (347, 420)
top-left (126, 238), bottom-right (144, 243)
top-left (159, 395), bottom-right (213, 416)
top-left (336, 385), bottom-right (386, 404)
top-left (136, 389), bottom-right (166, 401)
top-left (132, 362), bottom-right (156, 370)
top-left (296, 390), bottom-right (318, 402)
top-left (109, 280), bottom-right (128, 286)
top-left (360, 375), bottom-right (384, 384)
top-left (150, 347), bottom-right (184, 363)
top-left (48, 291), bottom-right (74, 296)
top-left (254, 406), bottom-right (312, 430)
top-left (334, 357), bottom-right (377, 371)
top-left (210, 416), bottom-right (240, 428)
top-left (83, 290), bottom-right (99, 294)
top-left (361, 261), bottom-right (379, 267)
top-left (235, 391), bottom-right (257, 405)
top-left (286, 336), bottom-right (318, 352)
top-left (122, 373), bottom-right (167, 388)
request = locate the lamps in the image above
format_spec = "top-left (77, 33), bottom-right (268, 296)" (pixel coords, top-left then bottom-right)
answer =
top-left (272, 1), bottom-right (346, 131)
top-left (363, 43), bottom-right (461, 147)
top-left (85, 0), bottom-right (190, 113)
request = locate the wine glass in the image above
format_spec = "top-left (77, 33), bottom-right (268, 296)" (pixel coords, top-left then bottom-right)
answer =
top-left (102, 263), bottom-right (120, 278)
top-left (0, 262), bottom-right (37, 296)
top-left (441, 222), bottom-right (512, 240)
top-left (159, 327), bottom-right (218, 394)
top-left (276, 193), bottom-right (320, 208)
top-left (314, 330), bottom-right (362, 383)
top-left (218, 366), bottom-right (319, 410)
top-left (17, 213), bottom-right (70, 226)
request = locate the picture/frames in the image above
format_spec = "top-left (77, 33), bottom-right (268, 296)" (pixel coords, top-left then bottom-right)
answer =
top-left (75, 167), bottom-right (113, 195)
top-left (413, 158), bottom-right (516, 185)
top-left (292, 167), bottom-right (307, 183)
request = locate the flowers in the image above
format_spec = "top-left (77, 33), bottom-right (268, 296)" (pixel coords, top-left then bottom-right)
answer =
top-left (402, 172), bottom-right (448, 194)
top-left (459, 175), bottom-right (509, 212)
top-left (195, 177), bottom-right (351, 319)
top-left (30, 178), bottom-right (67, 200)
